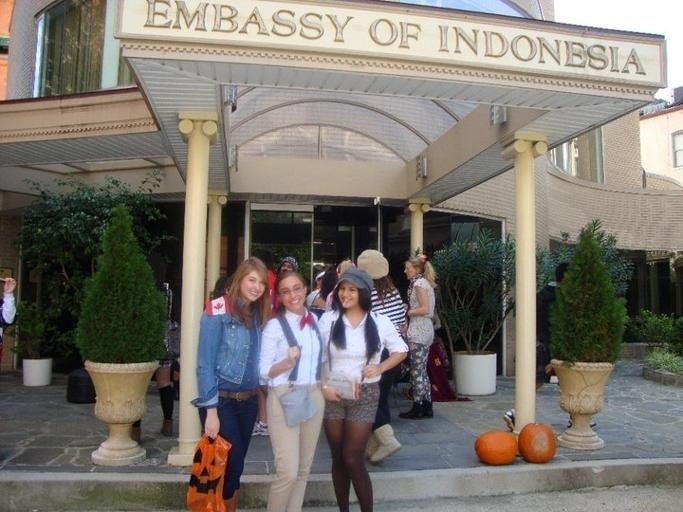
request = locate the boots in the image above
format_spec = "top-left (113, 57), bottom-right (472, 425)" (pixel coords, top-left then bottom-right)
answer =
top-left (131, 427), bottom-right (142, 444)
top-left (365, 436), bottom-right (380, 458)
top-left (161, 420), bottom-right (173, 436)
top-left (424, 400), bottom-right (434, 419)
top-left (369, 424), bottom-right (402, 464)
top-left (399, 402), bottom-right (424, 420)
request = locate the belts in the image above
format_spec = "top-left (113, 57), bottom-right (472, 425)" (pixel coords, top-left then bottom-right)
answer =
top-left (218, 388), bottom-right (256, 400)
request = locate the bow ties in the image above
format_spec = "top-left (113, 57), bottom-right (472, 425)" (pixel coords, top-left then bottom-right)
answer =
top-left (299, 314), bottom-right (316, 330)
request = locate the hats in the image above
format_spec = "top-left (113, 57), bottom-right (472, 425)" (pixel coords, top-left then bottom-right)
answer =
top-left (277, 256), bottom-right (299, 274)
top-left (358, 248), bottom-right (389, 281)
top-left (337, 260), bottom-right (356, 274)
top-left (333, 268), bottom-right (374, 296)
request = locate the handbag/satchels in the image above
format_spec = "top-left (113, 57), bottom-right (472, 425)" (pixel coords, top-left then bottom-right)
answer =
top-left (278, 387), bottom-right (318, 427)
top-left (325, 369), bottom-right (362, 401)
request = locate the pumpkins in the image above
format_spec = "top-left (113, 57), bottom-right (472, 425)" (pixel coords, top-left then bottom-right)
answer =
top-left (518, 422), bottom-right (556, 463)
top-left (476, 432), bottom-right (518, 463)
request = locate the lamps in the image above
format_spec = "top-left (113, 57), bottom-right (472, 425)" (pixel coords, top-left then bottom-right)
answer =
top-left (414, 151), bottom-right (427, 182)
top-left (226, 142), bottom-right (238, 171)
top-left (222, 83), bottom-right (239, 113)
top-left (486, 104), bottom-right (508, 127)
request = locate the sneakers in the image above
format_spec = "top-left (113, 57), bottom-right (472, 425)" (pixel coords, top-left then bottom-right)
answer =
top-left (251, 421), bottom-right (270, 437)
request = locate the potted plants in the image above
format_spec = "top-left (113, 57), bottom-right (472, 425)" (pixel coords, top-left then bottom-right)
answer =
top-left (5, 295), bottom-right (52, 385)
top-left (427, 226), bottom-right (516, 396)
top-left (17, 169), bottom-right (170, 405)
top-left (73, 199), bottom-right (168, 470)
top-left (543, 218), bottom-right (632, 454)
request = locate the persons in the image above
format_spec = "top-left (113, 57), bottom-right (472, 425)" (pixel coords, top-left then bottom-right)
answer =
top-left (503, 262), bottom-right (597, 431)
top-left (132, 278), bottom-right (181, 444)
top-left (0, 277), bottom-right (17, 359)
